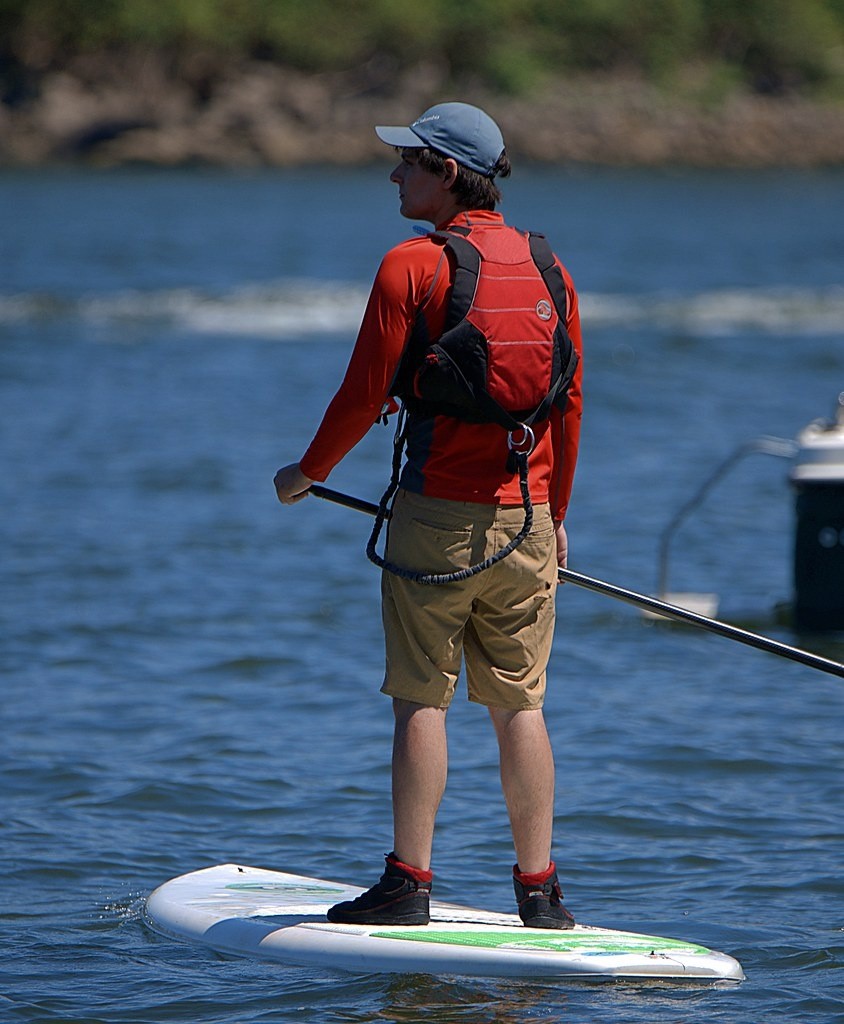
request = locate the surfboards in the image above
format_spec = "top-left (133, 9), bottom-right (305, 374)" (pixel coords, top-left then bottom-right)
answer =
top-left (141, 845), bottom-right (750, 996)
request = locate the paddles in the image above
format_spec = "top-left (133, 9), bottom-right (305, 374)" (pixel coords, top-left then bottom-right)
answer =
top-left (305, 479), bottom-right (844, 681)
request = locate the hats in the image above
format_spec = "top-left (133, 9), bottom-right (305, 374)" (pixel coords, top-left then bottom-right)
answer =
top-left (374, 102), bottom-right (506, 179)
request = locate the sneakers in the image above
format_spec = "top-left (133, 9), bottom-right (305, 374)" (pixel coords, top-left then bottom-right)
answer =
top-left (513, 861), bottom-right (575, 929)
top-left (327, 851), bottom-right (433, 924)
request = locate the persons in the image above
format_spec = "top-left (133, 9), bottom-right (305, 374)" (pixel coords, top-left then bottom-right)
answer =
top-left (274, 103), bottom-right (584, 932)
